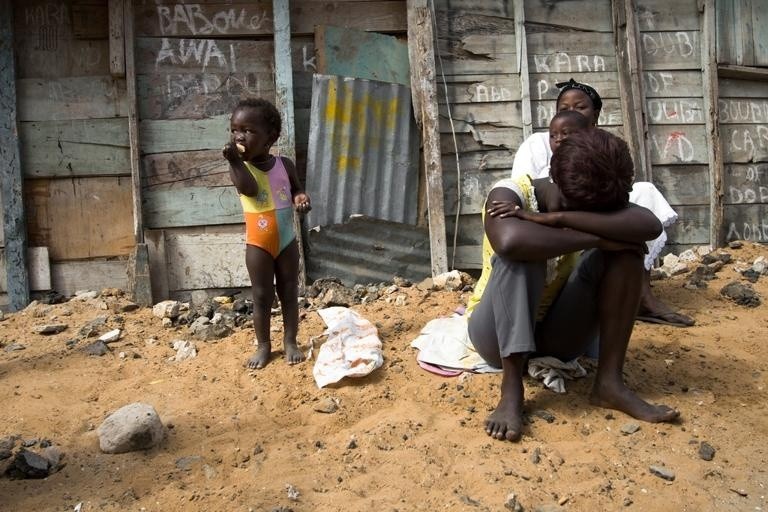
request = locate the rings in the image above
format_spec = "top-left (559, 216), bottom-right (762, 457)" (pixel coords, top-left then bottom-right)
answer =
top-left (514, 205), bottom-right (520, 211)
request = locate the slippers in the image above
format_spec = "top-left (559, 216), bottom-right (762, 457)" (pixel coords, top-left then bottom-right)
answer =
top-left (636, 311), bottom-right (697, 328)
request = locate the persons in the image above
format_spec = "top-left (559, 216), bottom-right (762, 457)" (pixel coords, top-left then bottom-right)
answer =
top-left (221, 97), bottom-right (313, 370)
top-left (462, 126), bottom-right (682, 442)
top-left (548, 109), bottom-right (594, 153)
top-left (509, 77), bottom-right (697, 328)
